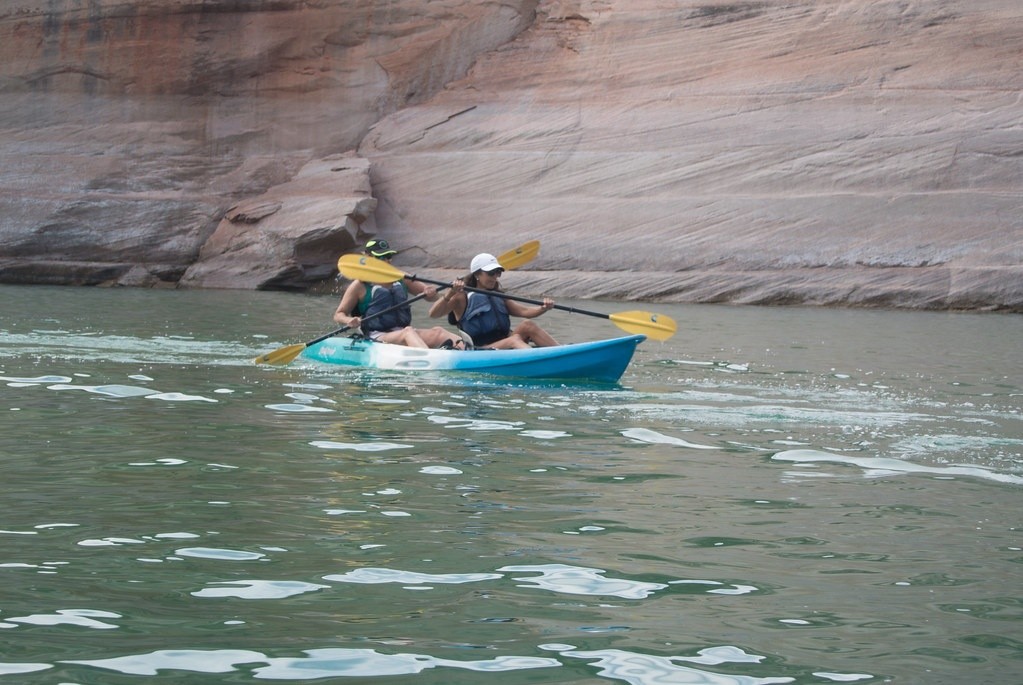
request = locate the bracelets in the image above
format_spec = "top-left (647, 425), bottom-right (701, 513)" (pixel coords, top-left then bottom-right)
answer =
top-left (443, 295), bottom-right (449, 302)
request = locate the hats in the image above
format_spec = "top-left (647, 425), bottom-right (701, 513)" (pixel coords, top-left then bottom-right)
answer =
top-left (470, 253), bottom-right (505, 274)
top-left (365, 239), bottom-right (398, 256)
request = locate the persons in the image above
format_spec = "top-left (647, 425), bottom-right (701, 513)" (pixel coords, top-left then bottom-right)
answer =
top-left (430, 253), bottom-right (559, 349)
top-left (334, 239), bottom-right (465, 349)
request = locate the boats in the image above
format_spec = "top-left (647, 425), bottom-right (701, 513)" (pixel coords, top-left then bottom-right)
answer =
top-left (295, 333), bottom-right (648, 385)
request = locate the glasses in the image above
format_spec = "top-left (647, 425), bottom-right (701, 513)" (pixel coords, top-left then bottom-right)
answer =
top-left (481, 269), bottom-right (502, 277)
top-left (374, 253), bottom-right (393, 260)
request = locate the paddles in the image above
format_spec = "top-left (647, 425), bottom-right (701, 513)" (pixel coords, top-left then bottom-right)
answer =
top-left (337, 252), bottom-right (678, 343)
top-left (254, 239), bottom-right (542, 369)
